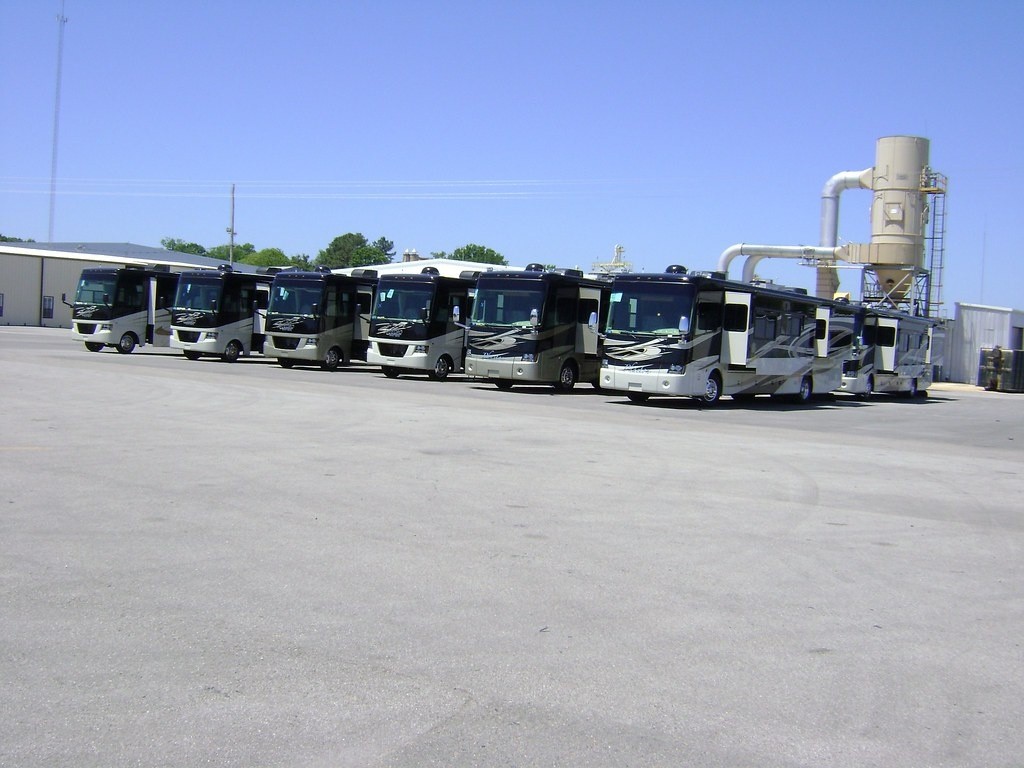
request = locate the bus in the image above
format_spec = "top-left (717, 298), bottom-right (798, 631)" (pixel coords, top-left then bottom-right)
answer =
top-left (355, 265), bottom-right (503, 382)
top-left (252, 265), bottom-right (386, 372)
top-left (833, 299), bottom-right (939, 402)
top-left (61, 264), bottom-right (191, 354)
top-left (160, 263), bottom-right (289, 364)
top-left (587, 265), bottom-right (842, 410)
top-left (452, 262), bottom-right (637, 394)
